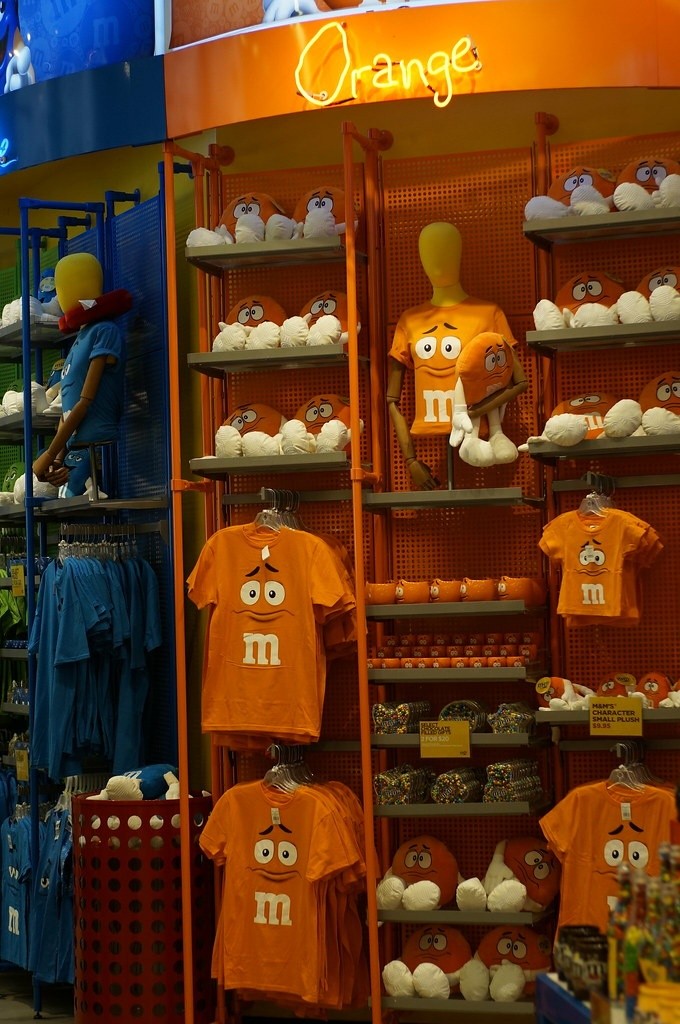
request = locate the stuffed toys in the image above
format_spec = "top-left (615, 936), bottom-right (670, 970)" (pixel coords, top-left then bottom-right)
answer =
top-left (539, 671), bottom-right (680, 712)
top-left (518, 154), bottom-right (680, 452)
top-left (0, 263), bottom-right (108, 507)
top-left (185, 186), bottom-right (363, 456)
top-left (449, 332), bottom-right (520, 467)
top-left (374, 831), bottom-right (563, 1004)
top-left (78, 762), bottom-right (212, 846)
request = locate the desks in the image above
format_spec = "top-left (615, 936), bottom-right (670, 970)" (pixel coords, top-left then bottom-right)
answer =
top-left (534, 972), bottom-right (590, 1024)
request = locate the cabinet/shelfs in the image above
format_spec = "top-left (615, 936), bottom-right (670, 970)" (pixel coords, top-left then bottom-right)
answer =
top-left (0, 118), bottom-right (680, 1024)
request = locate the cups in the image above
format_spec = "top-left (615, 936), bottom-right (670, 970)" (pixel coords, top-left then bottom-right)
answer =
top-left (366, 633), bottom-right (541, 669)
top-left (365, 575), bottom-right (546, 610)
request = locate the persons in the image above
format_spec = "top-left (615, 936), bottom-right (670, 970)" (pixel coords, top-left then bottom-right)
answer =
top-left (30, 252), bottom-right (131, 500)
top-left (384, 222), bottom-right (528, 491)
